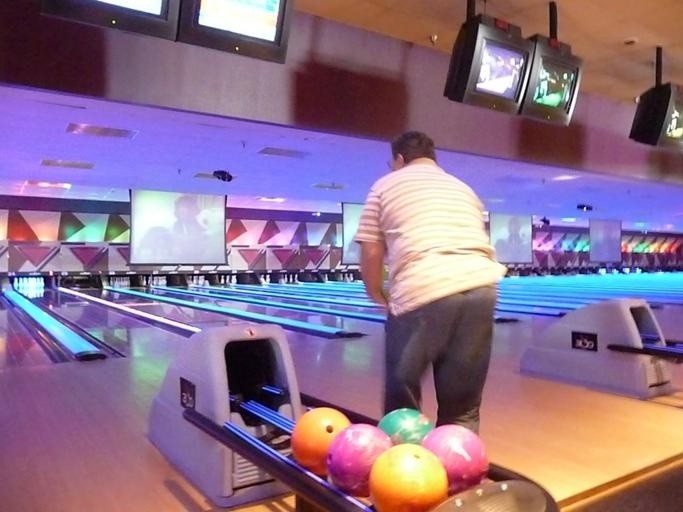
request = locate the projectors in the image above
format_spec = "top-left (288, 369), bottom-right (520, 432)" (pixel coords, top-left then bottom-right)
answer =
top-left (577, 205), bottom-right (592, 210)
top-left (213, 170), bottom-right (232, 182)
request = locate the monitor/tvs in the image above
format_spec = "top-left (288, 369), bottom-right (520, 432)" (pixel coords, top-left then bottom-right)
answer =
top-left (443, 13), bottom-right (534, 117)
top-left (178, 0), bottom-right (295, 66)
top-left (11, 0), bottom-right (181, 44)
top-left (628, 82), bottom-right (683, 148)
top-left (518, 32), bottom-right (584, 127)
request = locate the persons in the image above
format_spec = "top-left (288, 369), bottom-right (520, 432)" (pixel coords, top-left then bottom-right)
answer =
top-left (355, 130), bottom-right (510, 434)
